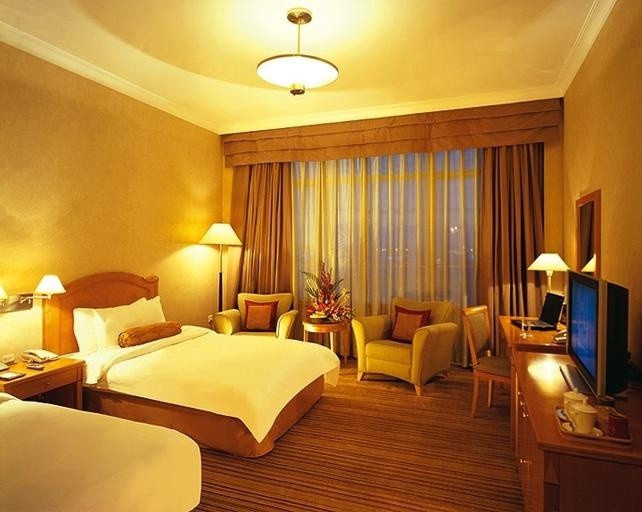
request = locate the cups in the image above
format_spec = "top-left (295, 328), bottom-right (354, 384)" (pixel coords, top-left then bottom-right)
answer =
top-left (562, 388), bottom-right (588, 415)
top-left (568, 400), bottom-right (597, 433)
top-left (608, 412), bottom-right (628, 437)
top-left (594, 393), bottom-right (617, 407)
top-left (522, 318), bottom-right (536, 337)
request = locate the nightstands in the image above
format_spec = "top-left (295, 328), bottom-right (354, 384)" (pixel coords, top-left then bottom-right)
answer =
top-left (0, 354), bottom-right (87, 411)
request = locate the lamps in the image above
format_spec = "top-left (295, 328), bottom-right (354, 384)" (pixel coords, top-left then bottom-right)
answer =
top-left (255, 7), bottom-right (340, 97)
top-left (526, 251), bottom-right (571, 291)
top-left (22, 274), bottom-right (68, 303)
top-left (0, 284), bottom-right (10, 308)
top-left (198, 222), bottom-right (244, 312)
top-left (580, 253), bottom-right (595, 273)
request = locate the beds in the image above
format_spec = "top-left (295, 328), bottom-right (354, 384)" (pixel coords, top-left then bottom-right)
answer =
top-left (1, 390), bottom-right (202, 511)
top-left (42, 271), bottom-right (342, 459)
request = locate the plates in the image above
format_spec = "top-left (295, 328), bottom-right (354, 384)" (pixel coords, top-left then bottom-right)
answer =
top-left (562, 422), bottom-right (602, 439)
top-left (555, 408), bottom-right (568, 421)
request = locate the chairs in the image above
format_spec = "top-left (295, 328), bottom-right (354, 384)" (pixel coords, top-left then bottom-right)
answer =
top-left (350, 296), bottom-right (458, 396)
top-left (461, 304), bottom-right (510, 418)
top-left (213, 292), bottom-right (298, 339)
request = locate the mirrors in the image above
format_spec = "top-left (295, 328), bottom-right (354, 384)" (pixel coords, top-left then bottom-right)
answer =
top-left (575, 188), bottom-right (602, 276)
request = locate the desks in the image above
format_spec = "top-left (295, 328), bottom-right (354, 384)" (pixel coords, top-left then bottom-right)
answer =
top-left (498, 313), bottom-right (567, 391)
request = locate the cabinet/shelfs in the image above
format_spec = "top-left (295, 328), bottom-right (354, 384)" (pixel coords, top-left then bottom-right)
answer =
top-left (510, 350), bottom-right (642, 511)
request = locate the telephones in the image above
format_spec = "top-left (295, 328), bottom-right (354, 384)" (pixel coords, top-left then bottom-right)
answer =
top-left (552, 329), bottom-right (567, 344)
top-left (21, 349), bottom-right (58, 364)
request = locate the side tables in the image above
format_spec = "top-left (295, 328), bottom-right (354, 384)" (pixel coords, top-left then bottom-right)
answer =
top-left (302, 317), bottom-right (350, 364)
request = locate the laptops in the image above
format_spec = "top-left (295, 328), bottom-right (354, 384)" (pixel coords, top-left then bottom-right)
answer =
top-left (511, 292), bottom-right (564, 330)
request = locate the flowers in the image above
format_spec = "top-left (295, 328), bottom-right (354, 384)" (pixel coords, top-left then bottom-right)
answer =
top-left (298, 263), bottom-right (354, 322)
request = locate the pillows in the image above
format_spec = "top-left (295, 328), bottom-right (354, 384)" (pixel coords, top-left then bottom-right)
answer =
top-left (94, 295), bottom-right (167, 349)
top-left (72, 296), bottom-right (147, 353)
top-left (387, 304), bottom-right (432, 344)
top-left (118, 319), bottom-right (183, 349)
top-left (240, 299), bottom-right (279, 332)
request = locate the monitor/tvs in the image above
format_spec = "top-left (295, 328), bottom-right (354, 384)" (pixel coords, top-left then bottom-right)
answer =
top-left (560, 270), bottom-right (629, 402)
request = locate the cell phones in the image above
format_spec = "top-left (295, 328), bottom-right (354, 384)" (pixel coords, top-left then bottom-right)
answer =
top-left (27, 364), bottom-right (44, 370)
